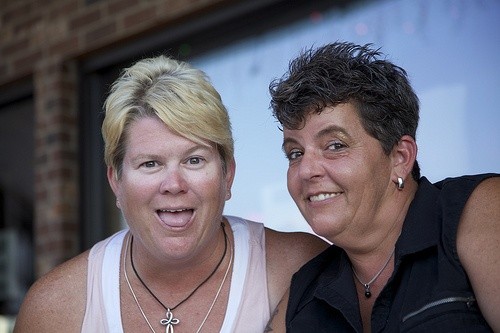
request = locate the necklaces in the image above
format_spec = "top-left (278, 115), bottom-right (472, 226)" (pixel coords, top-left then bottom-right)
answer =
top-left (352, 249), bottom-right (395, 298)
top-left (123, 222), bottom-right (233, 333)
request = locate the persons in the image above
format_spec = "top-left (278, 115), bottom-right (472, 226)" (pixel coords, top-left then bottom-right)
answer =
top-left (13, 56), bottom-right (332, 333)
top-left (269, 42), bottom-right (500, 333)
